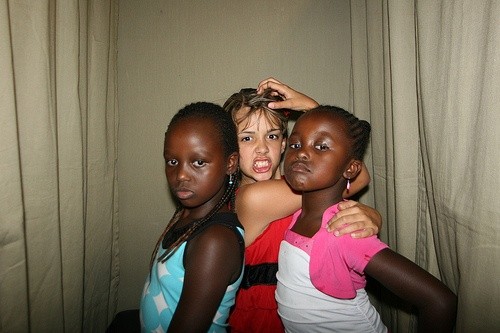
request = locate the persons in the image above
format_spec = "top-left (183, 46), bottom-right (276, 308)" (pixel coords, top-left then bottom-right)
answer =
top-left (139, 102), bottom-right (246, 333)
top-left (223, 77), bottom-right (383, 333)
top-left (274, 105), bottom-right (459, 333)
